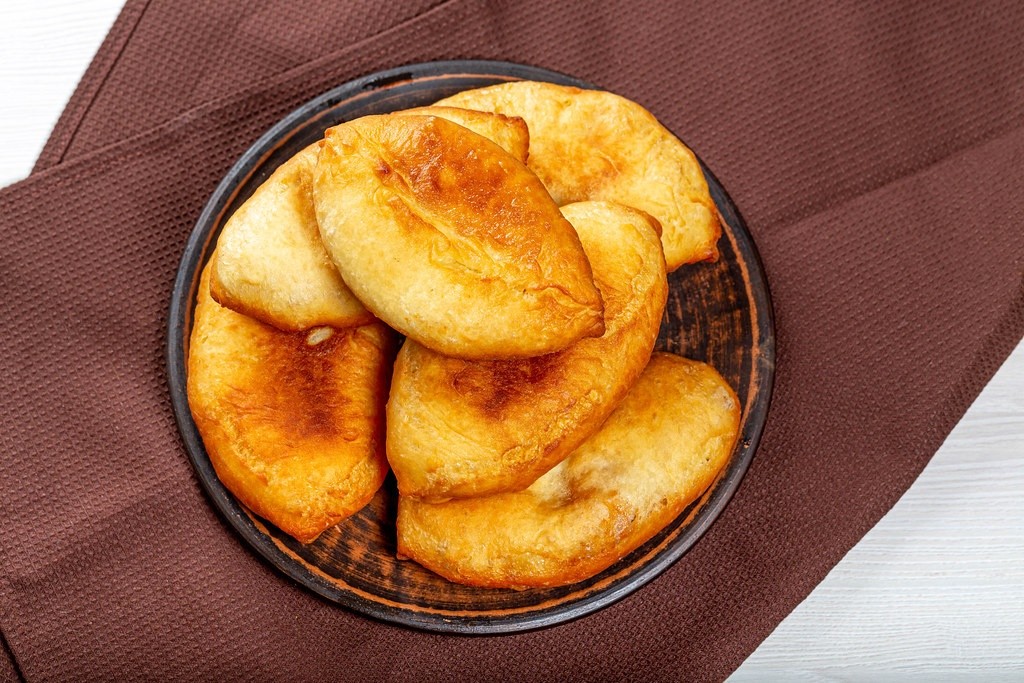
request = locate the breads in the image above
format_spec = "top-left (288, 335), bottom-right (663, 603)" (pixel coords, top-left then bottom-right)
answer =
top-left (186, 80), bottom-right (741, 591)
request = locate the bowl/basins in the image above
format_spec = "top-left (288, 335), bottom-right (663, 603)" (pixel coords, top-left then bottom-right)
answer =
top-left (166, 61), bottom-right (777, 639)
